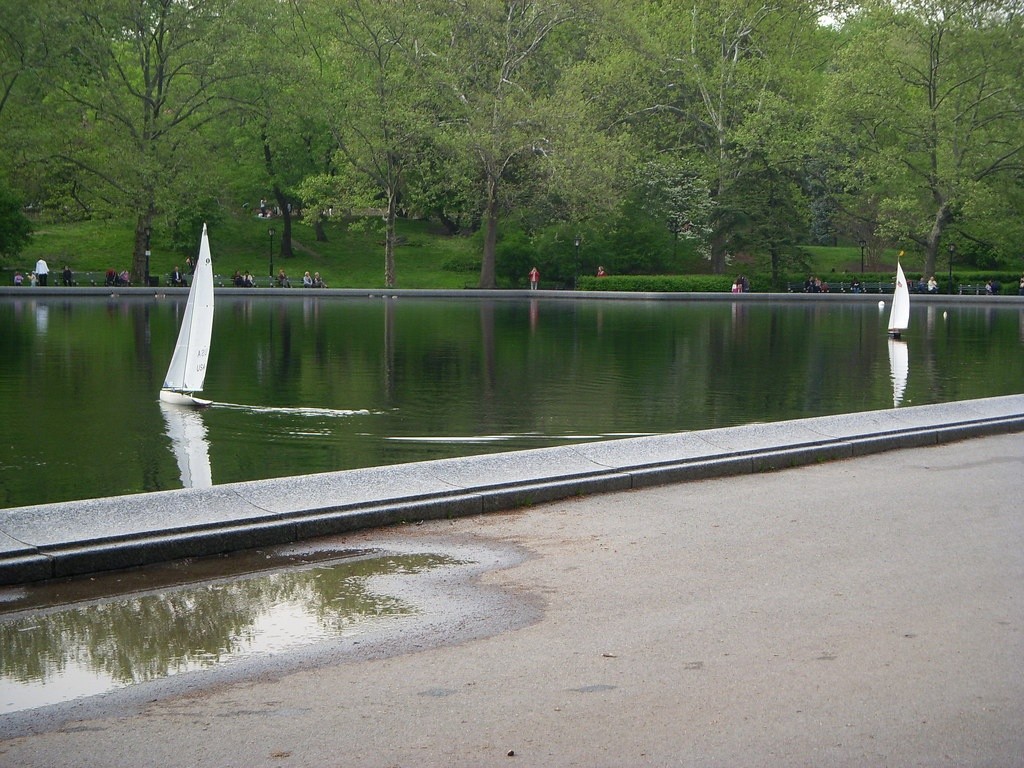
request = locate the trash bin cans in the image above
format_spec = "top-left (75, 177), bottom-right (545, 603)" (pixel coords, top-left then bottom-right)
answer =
top-left (148, 276), bottom-right (159, 287)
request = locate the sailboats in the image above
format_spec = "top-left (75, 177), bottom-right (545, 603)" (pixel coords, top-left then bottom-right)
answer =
top-left (887, 336), bottom-right (909, 408)
top-left (160, 399), bottom-right (213, 489)
top-left (887, 256), bottom-right (910, 339)
top-left (158, 222), bottom-right (216, 406)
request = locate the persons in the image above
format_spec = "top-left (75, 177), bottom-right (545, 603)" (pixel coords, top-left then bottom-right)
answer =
top-left (852, 277), bottom-right (860, 293)
top-left (119, 269), bottom-right (129, 286)
top-left (234, 269), bottom-right (252, 288)
top-left (805, 276), bottom-right (831, 293)
top-left (529, 267), bottom-right (539, 290)
top-left (907, 276), bottom-right (939, 294)
top-left (598, 266), bottom-right (607, 277)
top-left (260, 197), bottom-right (334, 217)
top-left (62, 266), bottom-right (72, 287)
top-left (303, 271), bottom-right (323, 288)
top-left (14, 257), bottom-right (50, 287)
top-left (278, 269), bottom-right (289, 288)
top-left (173, 267), bottom-right (183, 287)
top-left (985, 280), bottom-right (993, 295)
top-left (732, 276), bottom-right (750, 293)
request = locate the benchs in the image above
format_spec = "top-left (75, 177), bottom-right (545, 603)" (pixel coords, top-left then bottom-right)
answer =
top-left (957, 283), bottom-right (986, 295)
top-left (824, 281), bottom-right (862, 293)
top-left (253, 275), bottom-right (281, 288)
top-left (165, 273), bottom-right (217, 287)
top-left (913, 280), bottom-right (941, 294)
top-left (862, 282), bottom-right (896, 294)
top-left (287, 277), bottom-right (324, 288)
top-left (787, 281), bottom-right (824, 293)
top-left (36, 271), bottom-right (131, 287)
top-left (217, 273), bottom-right (234, 287)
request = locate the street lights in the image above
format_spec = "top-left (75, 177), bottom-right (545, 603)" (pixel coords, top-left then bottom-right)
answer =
top-left (859, 241), bottom-right (866, 272)
top-left (144, 227), bottom-right (154, 287)
top-left (948, 244), bottom-right (955, 295)
top-left (268, 228), bottom-right (276, 277)
top-left (573, 238), bottom-right (582, 290)
